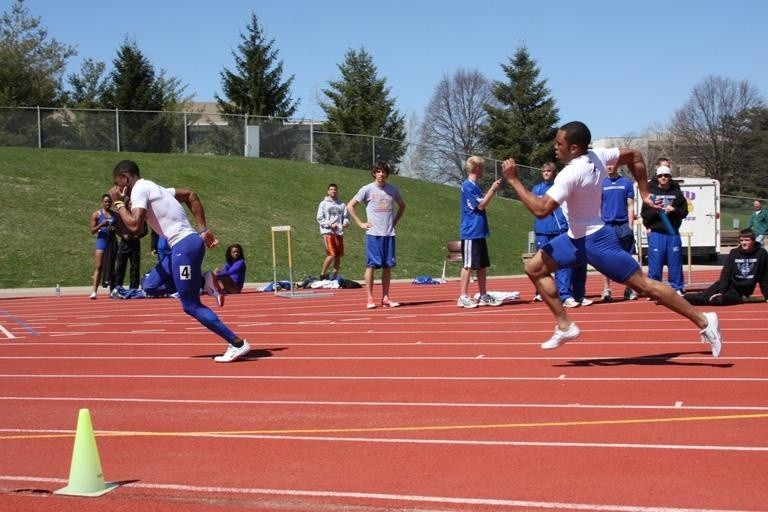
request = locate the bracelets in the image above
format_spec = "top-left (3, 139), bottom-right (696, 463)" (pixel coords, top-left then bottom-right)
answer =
top-left (488, 187), bottom-right (495, 193)
top-left (112, 199), bottom-right (125, 212)
top-left (198, 225), bottom-right (209, 236)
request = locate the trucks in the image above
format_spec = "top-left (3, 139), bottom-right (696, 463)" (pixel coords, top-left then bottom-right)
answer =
top-left (633, 176), bottom-right (721, 266)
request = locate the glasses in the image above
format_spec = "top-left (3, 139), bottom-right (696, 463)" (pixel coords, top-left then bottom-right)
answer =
top-left (657, 174), bottom-right (669, 178)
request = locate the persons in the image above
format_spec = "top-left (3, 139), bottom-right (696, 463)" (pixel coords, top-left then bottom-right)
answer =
top-left (211, 242), bottom-right (247, 294)
top-left (107, 159), bottom-right (250, 364)
top-left (87, 194), bottom-right (116, 300)
top-left (109, 194), bottom-right (149, 298)
top-left (315, 182), bottom-right (350, 282)
top-left (499, 119), bottom-right (724, 357)
top-left (455, 155), bottom-right (503, 309)
top-left (346, 160), bottom-right (407, 309)
top-left (150, 226), bottom-right (169, 262)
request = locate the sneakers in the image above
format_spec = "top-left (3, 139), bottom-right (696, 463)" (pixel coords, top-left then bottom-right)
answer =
top-left (202, 270), bottom-right (225, 307)
top-left (533, 293), bottom-right (544, 302)
top-left (562, 297), bottom-right (580, 308)
top-left (698, 311), bottom-right (723, 358)
top-left (89, 290), bottom-right (97, 299)
top-left (456, 296), bottom-right (479, 309)
top-left (366, 299), bottom-right (376, 309)
top-left (541, 320), bottom-right (581, 351)
top-left (624, 288), bottom-right (638, 300)
top-left (601, 288), bottom-right (612, 301)
top-left (214, 338), bottom-right (251, 363)
top-left (579, 297), bottom-right (594, 306)
top-left (382, 300), bottom-right (401, 308)
top-left (478, 294), bottom-right (504, 307)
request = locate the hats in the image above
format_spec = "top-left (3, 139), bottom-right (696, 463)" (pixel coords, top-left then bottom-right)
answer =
top-left (656, 166), bottom-right (672, 176)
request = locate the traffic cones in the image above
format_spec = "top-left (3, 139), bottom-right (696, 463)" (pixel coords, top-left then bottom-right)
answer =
top-left (52, 407), bottom-right (120, 497)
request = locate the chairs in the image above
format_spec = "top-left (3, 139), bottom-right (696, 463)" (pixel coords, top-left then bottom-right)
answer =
top-left (440, 238), bottom-right (464, 283)
